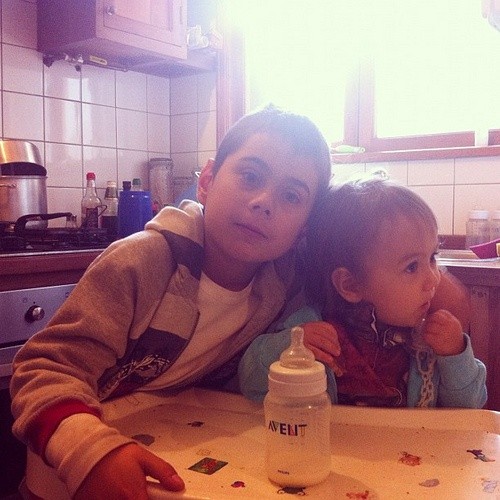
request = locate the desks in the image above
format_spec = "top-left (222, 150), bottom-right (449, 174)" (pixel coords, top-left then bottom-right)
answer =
top-left (55, 390), bottom-right (500, 500)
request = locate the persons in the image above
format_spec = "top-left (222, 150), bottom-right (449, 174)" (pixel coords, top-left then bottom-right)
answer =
top-left (12, 107), bottom-right (471, 500)
top-left (236, 177), bottom-right (488, 407)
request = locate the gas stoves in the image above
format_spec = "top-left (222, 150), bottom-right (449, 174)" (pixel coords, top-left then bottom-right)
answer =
top-left (1, 229), bottom-right (123, 345)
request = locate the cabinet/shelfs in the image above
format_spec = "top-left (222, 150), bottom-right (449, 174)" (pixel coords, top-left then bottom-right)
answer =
top-left (431, 267), bottom-right (500, 410)
top-left (37, 0), bottom-right (214, 79)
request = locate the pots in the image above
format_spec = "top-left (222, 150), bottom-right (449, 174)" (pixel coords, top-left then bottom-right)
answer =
top-left (0, 140), bottom-right (49, 232)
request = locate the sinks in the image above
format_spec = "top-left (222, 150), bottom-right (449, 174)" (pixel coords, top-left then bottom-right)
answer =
top-left (433, 249), bottom-right (500, 262)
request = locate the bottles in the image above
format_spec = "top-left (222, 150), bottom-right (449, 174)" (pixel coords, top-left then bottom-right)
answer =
top-left (491, 210), bottom-right (500, 240)
top-left (102, 180), bottom-right (119, 233)
top-left (66, 214), bottom-right (78, 229)
top-left (148, 158), bottom-right (175, 203)
top-left (464, 210), bottom-right (489, 249)
top-left (123, 179), bottom-right (143, 191)
top-left (81, 173), bottom-right (101, 228)
top-left (264, 326), bottom-right (336, 485)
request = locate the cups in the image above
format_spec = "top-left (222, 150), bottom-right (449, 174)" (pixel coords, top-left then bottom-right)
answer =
top-left (117, 191), bottom-right (155, 238)
